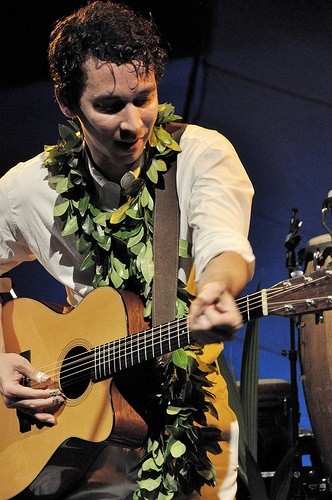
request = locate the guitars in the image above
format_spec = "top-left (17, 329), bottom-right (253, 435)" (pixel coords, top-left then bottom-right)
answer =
top-left (1, 248), bottom-right (332, 500)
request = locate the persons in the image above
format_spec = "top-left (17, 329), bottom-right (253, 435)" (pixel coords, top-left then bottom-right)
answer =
top-left (0, 0), bottom-right (255, 500)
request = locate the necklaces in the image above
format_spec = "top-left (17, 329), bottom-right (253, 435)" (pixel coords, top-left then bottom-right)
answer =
top-left (42, 101), bottom-right (222, 500)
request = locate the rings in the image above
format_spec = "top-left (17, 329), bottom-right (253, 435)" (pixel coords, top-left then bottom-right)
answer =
top-left (36, 372), bottom-right (44, 384)
top-left (49, 388), bottom-right (60, 406)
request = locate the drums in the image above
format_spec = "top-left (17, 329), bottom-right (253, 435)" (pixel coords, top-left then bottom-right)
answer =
top-left (300, 233), bottom-right (332, 477)
top-left (231, 376), bottom-right (298, 500)
